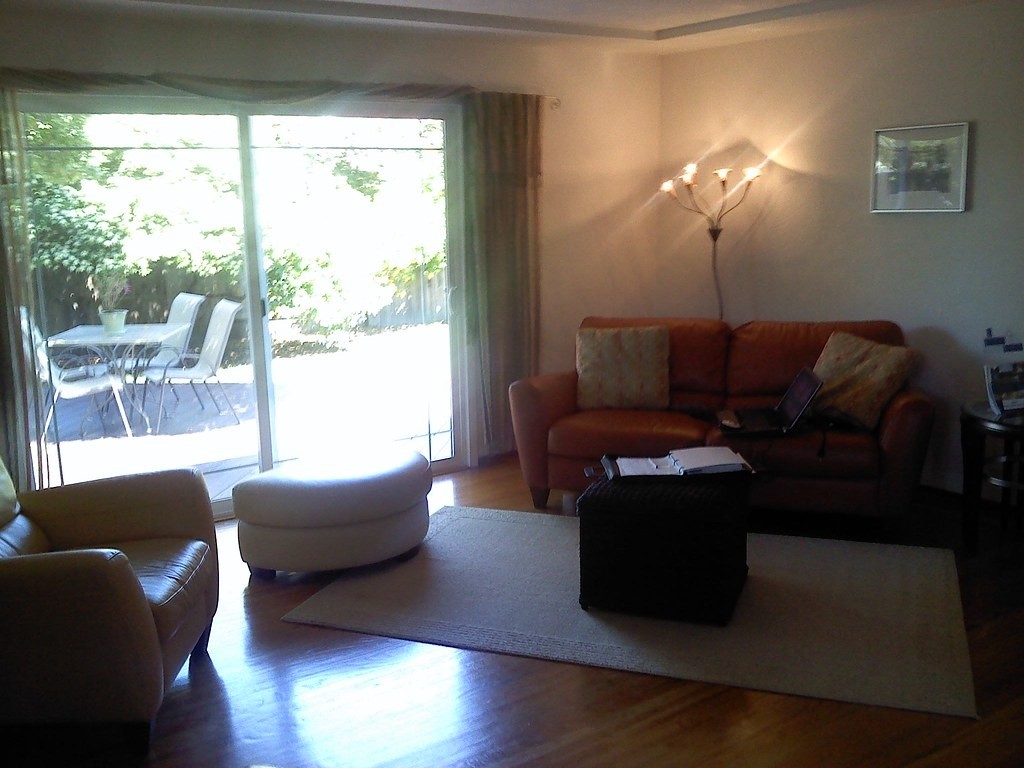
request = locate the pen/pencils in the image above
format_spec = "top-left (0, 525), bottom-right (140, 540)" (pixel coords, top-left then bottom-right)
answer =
top-left (648, 458), bottom-right (659, 468)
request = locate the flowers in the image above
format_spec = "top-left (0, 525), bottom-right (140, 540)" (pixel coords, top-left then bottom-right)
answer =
top-left (87, 263), bottom-right (132, 311)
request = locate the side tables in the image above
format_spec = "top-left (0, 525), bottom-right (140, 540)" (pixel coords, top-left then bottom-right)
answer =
top-left (959, 399), bottom-right (1024, 553)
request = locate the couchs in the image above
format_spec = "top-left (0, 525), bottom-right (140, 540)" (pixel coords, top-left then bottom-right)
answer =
top-left (0, 457), bottom-right (220, 767)
top-left (509, 315), bottom-right (935, 540)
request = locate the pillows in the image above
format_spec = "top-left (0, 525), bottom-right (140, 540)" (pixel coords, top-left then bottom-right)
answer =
top-left (800, 330), bottom-right (918, 430)
top-left (575, 324), bottom-right (671, 412)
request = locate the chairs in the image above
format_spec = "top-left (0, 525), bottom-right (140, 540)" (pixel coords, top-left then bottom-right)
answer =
top-left (14, 305), bottom-right (109, 437)
top-left (140, 298), bottom-right (242, 433)
top-left (111, 291), bottom-right (207, 409)
top-left (21, 321), bottom-right (133, 442)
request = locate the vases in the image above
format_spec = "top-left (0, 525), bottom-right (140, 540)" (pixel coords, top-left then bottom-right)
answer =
top-left (99, 308), bottom-right (129, 335)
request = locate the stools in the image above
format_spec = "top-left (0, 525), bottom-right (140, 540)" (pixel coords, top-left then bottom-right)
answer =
top-left (233, 444), bottom-right (434, 578)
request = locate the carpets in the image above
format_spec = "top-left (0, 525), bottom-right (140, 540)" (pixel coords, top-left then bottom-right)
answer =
top-left (280, 503), bottom-right (980, 722)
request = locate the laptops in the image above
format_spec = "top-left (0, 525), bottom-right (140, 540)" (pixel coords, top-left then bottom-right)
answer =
top-left (716, 367), bottom-right (823, 439)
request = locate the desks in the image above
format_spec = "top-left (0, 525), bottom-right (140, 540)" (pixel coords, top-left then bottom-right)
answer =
top-left (48, 320), bottom-right (192, 439)
top-left (577, 456), bottom-right (748, 626)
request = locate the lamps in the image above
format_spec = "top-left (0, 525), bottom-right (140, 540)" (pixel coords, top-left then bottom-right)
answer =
top-left (657, 163), bottom-right (762, 319)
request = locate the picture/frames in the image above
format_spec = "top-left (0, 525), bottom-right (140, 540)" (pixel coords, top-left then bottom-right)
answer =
top-left (870, 121), bottom-right (968, 213)
